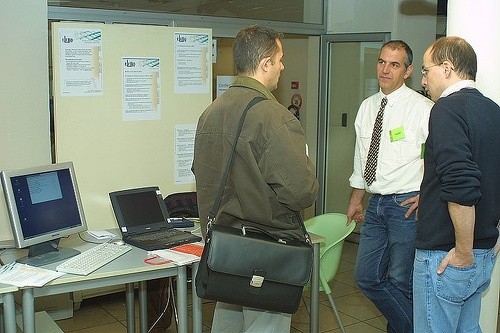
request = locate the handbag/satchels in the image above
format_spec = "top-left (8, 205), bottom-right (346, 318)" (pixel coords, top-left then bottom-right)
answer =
top-left (195, 224), bottom-right (314, 314)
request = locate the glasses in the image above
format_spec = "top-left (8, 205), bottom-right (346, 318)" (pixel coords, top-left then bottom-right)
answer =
top-left (420, 63), bottom-right (454, 77)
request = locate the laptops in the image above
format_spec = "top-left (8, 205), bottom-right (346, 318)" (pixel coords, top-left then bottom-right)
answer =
top-left (108, 186), bottom-right (203, 251)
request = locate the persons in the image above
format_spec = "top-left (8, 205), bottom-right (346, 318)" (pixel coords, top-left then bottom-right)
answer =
top-left (191, 24), bottom-right (319, 333)
top-left (347, 40), bottom-right (435, 333)
top-left (412, 36), bottom-right (499, 333)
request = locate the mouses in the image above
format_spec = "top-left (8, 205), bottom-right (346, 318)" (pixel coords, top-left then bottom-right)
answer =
top-left (108, 239), bottom-right (126, 245)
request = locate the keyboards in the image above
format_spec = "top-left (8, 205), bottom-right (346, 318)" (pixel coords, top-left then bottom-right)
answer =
top-left (55, 242), bottom-right (132, 276)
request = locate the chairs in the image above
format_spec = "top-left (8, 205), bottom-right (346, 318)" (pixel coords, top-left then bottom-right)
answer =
top-left (303, 212), bottom-right (356, 333)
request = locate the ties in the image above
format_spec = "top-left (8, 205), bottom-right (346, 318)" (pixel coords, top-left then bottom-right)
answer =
top-left (364, 97), bottom-right (388, 186)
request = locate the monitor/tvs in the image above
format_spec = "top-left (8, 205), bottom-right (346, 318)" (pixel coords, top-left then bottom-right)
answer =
top-left (0, 161), bottom-right (88, 267)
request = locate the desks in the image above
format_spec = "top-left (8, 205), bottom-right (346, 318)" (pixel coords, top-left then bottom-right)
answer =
top-left (0, 221), bottom-right (327, 333)
